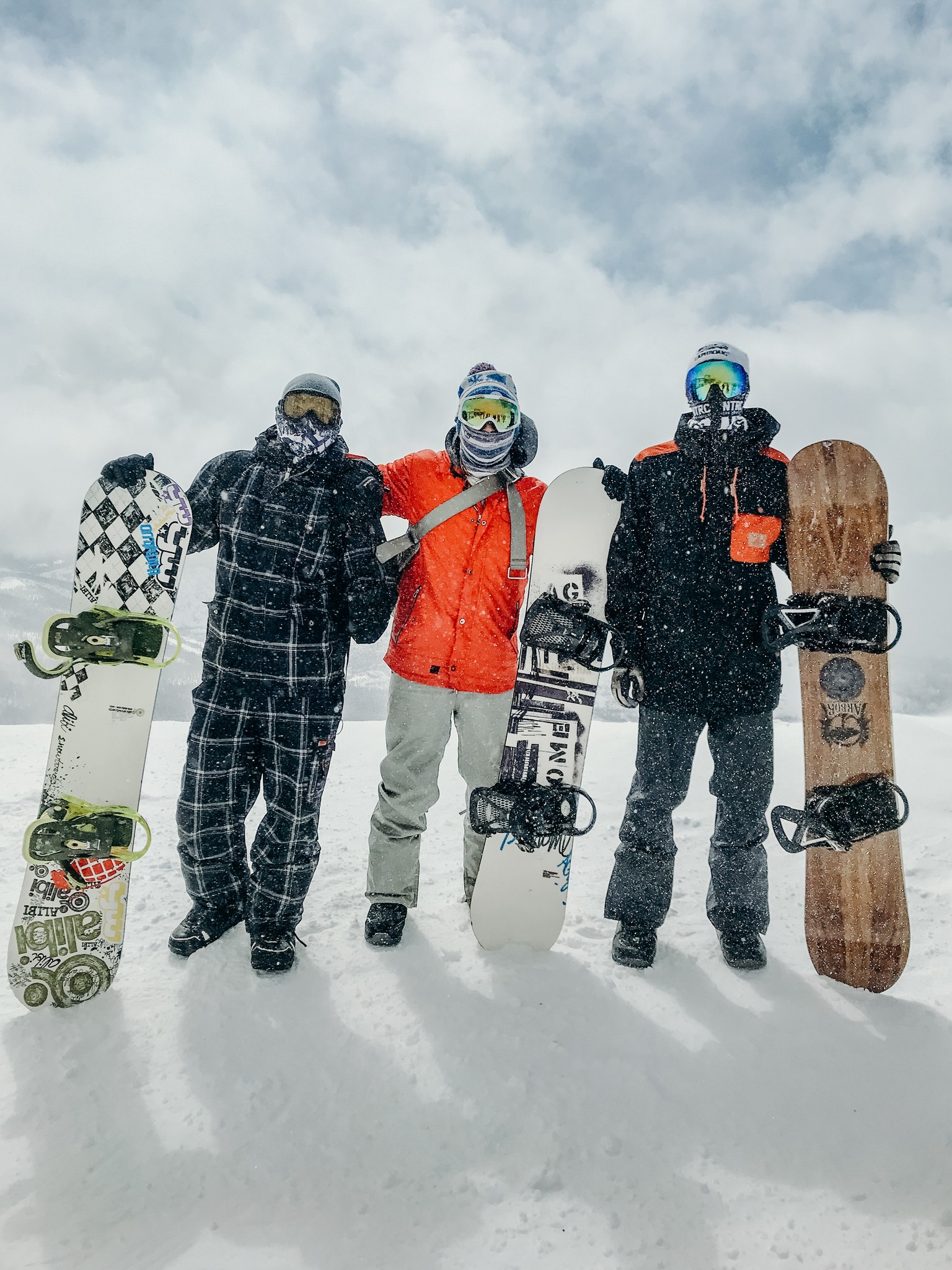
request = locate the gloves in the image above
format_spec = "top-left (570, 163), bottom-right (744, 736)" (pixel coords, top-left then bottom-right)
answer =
top-left (99, 452), bottom-right (153, 488)
top-left (593, 457), bottom-right (628, 502)
top-left (610, 664), bottom-right (643, 708)
top-left (870, 539), bottom-right (901, 584)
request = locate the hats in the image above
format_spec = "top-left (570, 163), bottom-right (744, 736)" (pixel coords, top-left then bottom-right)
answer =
top-left (686, 342), bottom-right (750, 401)
top-left (456, 362), bottom-right (518, 419)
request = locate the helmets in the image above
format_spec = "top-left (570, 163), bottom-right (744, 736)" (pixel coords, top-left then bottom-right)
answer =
top-left (278, 374), bottom-right (343, 455)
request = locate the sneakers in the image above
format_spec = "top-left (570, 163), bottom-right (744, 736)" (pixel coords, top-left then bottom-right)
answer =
top-left (167, 901), bottom-right (239, 956)
top-left (713, 923), bottom-right (766, 970)
top-left (364, 902), bottom-right (407, 946)
top-left (612, 918), bottom-right (657, 970)
top-left (248, 925), bottom-right (307, 973)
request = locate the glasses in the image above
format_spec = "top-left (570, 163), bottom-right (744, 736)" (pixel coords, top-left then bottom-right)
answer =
top-left (282, 388), bottom-right (337, 425)
top-left (685, 361), bottom-right (751, 405)
top-left (458, 398), bottom-right (521, 433)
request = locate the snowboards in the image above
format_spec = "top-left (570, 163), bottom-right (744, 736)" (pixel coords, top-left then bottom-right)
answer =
top-left (787, 439), bottom-right (910, 995)
top-left (6, 469), bottom-right (193, 1013)
top-left (471, 468), bottom-right (624, 956)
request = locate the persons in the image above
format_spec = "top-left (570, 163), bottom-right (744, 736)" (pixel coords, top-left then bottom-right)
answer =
top-left (604, 341), bottom-right (900, 969)
top-left (346, 361), bottom-right (627, 948)
top-left (100, 374), bottom-right (401, 977)
